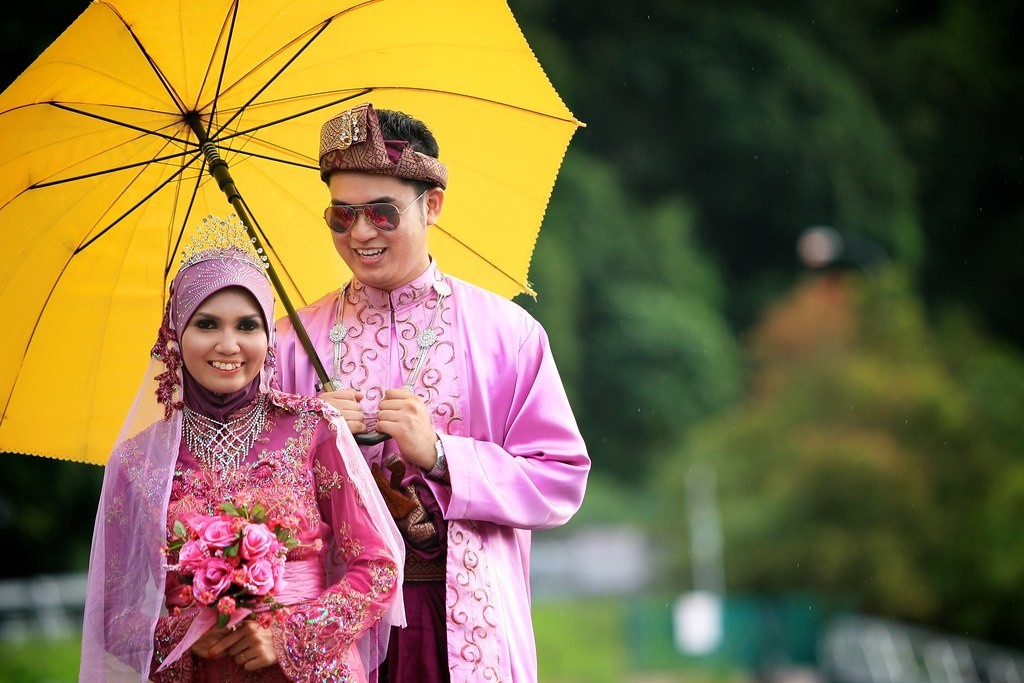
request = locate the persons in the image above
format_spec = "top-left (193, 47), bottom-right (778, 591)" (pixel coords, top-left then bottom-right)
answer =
top-left (266, 101), bottom-right (586, 683)
top-left (87, 247), bottom-right (401, 683)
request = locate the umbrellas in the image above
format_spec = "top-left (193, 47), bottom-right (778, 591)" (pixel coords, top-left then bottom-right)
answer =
top-left (0, 0), bottom-right (585, 468)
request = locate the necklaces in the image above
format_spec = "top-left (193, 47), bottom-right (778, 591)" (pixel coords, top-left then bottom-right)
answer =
top-left (180, 392), bottom-right (265, 483)
top-left (329, 276), bottom-right (453, 421)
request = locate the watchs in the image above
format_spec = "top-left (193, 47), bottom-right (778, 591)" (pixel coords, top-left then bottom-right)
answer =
top-left (427, 438), bottom-right (449, 480)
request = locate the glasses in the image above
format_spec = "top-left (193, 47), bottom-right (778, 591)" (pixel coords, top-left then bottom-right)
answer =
top-left (323, 188), bottom-right (428, 233)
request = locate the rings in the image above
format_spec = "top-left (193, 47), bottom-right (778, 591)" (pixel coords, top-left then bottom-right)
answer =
top-left (241, 653), bottom-right (247, 663)
